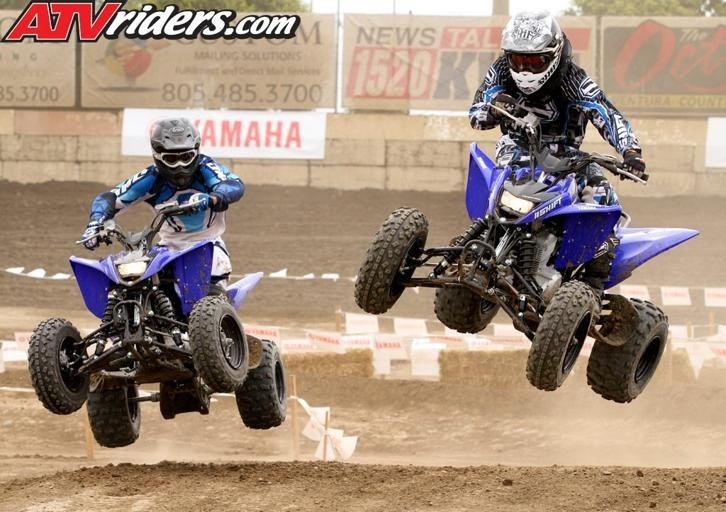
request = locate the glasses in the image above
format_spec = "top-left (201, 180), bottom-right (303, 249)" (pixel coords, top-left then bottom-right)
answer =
top-left (506, 44), bottom-right (559, 73)
top-left (151, 145), bottom-right (201, 171)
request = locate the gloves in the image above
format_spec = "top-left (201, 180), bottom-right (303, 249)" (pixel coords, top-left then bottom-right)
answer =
top-left (489, 92), bottom-right (515, 118)
top-left (620, 147), bottom-right (648, 183)
top-left (187, 192), bottom-right (215, 213)
top-left (81, 221), bottom-right (109, 251)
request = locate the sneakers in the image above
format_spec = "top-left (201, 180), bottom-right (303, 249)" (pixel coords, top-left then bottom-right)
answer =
top-left (574, 274), bottom-right (612, 323)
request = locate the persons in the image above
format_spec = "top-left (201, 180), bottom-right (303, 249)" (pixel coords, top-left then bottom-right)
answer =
top-left (468, 7), bottom-right (648, 320)
top-left (81, 117), bottom-right (244, 301)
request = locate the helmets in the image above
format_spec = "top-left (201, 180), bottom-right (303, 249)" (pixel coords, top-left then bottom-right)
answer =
top-left (148, 115), bottom-right (202, 189)
top-left (500, 7), bottom-right (565, 96)
